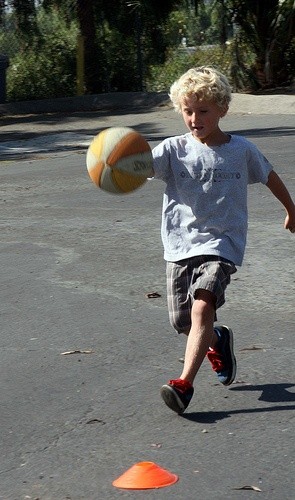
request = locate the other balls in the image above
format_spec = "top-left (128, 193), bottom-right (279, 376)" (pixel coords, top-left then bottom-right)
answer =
top-left (85, 127), bottom-right (153, 196)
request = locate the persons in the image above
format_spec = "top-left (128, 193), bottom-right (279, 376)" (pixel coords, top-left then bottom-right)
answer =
top-left (148, 65), bottom-right (295, 415)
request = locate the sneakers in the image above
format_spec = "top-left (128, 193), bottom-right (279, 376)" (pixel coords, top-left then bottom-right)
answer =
top-left (161, 378), bottom-right (194, 414)
top-left (206, 324), bottom-right (237, 385)
